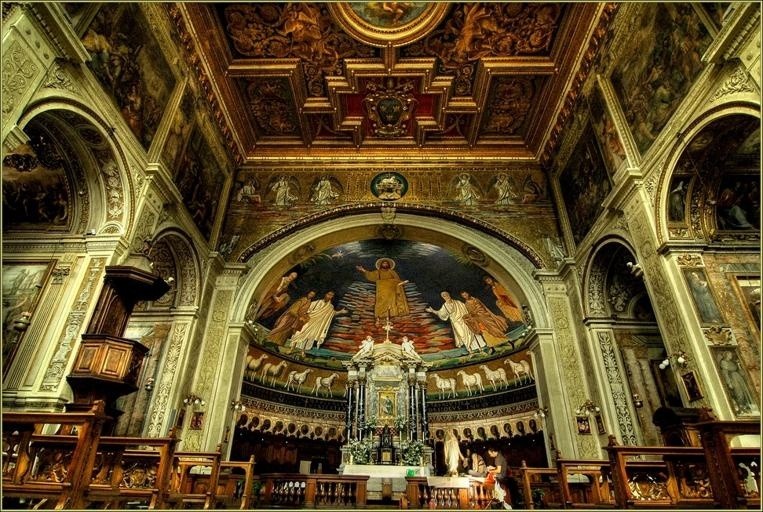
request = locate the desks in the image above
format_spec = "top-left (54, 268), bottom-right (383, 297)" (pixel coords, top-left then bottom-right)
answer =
top-left (259, 464), bottom-right (511, 511)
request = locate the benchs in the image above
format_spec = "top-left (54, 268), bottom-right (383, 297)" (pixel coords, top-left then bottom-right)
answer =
top-left (513, 409), bottom-right (760, 510)
top-left (0, 399), bottom-right (258, 511)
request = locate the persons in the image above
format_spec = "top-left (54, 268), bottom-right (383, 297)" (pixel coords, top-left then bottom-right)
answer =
top-left (481, 446), bottom-right (508, 484)
top-left (452, 173), bottom-right (480, 205)
top-left (463, 445), bottom-right (484, 478)
top-left (354, 256), bottom-right (412, 330)
top-left (271, 174), bottom-right (298, 208)
top-left (251, 272), bottom-right (525, 362)
top-left (689, 270), bottom-right (722, 327)
top-left (234, 179), bottom-right (262, 206)
top-left (382, 395), bottom-right (394, 415)
top-left (719, 350), bottom-right (757, 416)
top-left (492, 172), bottom-right (517, 213)
top-left (313, 175), bottom-right (341, 205)
top-left (440, 426), bottom-right (464, 477)
